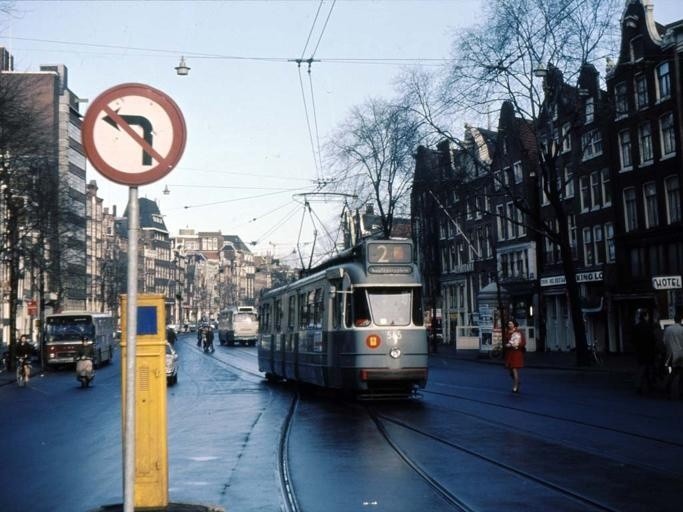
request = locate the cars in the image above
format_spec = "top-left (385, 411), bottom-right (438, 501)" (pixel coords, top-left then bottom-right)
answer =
top-left (165, 339), bottom-right (179, 384)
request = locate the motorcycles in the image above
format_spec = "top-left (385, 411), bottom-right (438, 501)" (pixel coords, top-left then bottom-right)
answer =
top-left (75, 355), bottom-right (95, 386)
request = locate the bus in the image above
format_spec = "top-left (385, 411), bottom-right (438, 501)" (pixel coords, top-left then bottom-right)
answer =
top-left (256, 237), bottom-right (430, 401)
top-left (217, 306), bottom-right (259, 345)
top-left (35, 311), bottom-right (114, 367)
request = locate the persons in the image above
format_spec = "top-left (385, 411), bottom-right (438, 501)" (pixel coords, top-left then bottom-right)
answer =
top-left (503, 321), bottom-right (526, 391)
top-left (634, 309), bottom-right (683, 402)
top-left (74, 334), bottom-right (93, 359)
top-left (167, 322), bottom-right (215, 351)
top-left (16, 334), bottom-right (31, 381)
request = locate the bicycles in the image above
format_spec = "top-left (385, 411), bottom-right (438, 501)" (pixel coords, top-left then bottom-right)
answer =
top-left (13, 355), bottom-right (31, 386)
